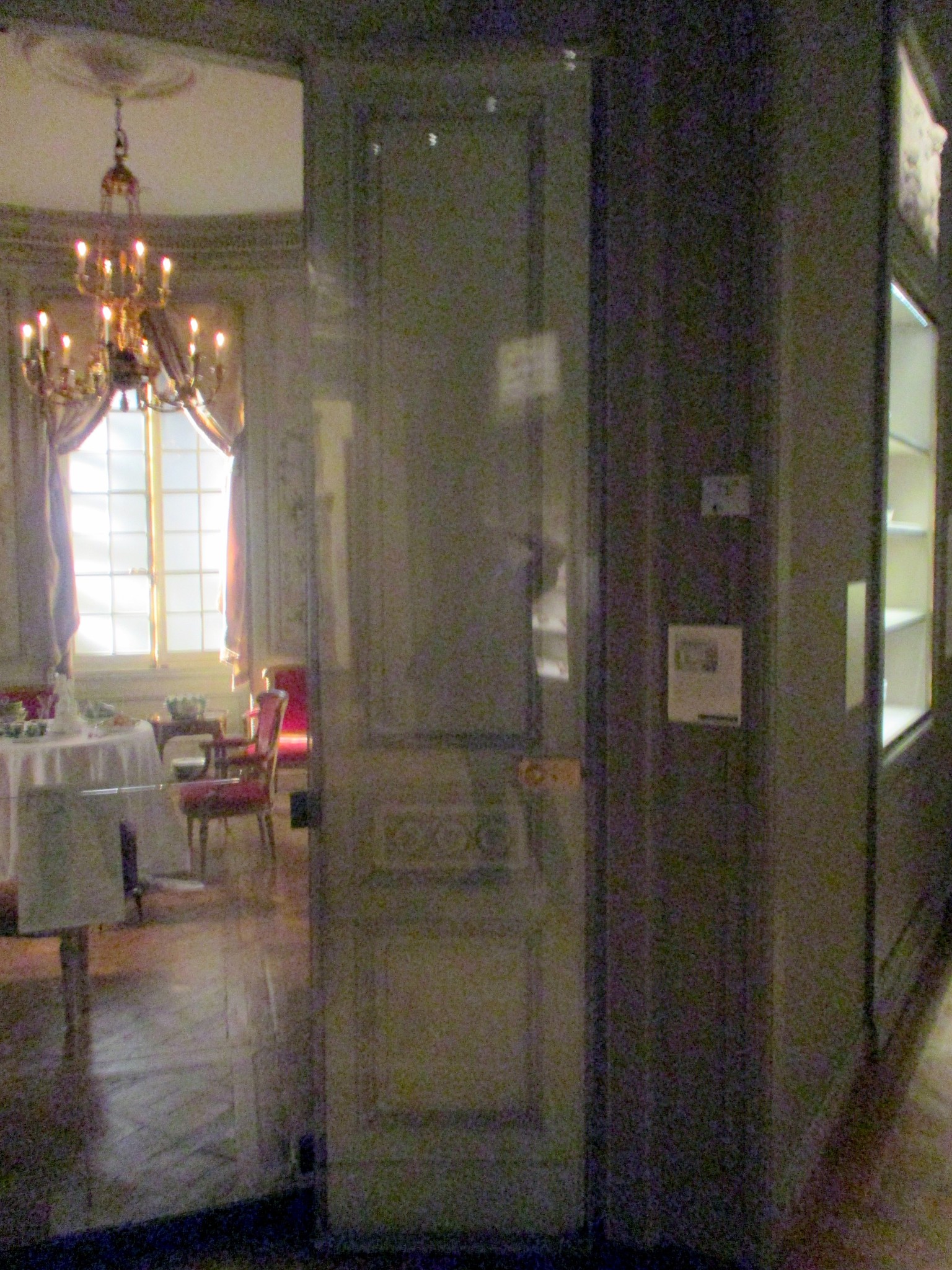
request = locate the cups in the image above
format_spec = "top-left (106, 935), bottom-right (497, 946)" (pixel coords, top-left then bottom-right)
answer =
top-left (0, 719), bottom-right (49, 738)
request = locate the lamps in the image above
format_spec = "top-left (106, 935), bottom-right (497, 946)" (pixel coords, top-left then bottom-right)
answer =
top-left (13, 18), bottom-right (228, 417)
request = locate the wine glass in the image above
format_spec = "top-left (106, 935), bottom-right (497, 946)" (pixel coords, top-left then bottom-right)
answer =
top-left (35, 693), bottom-right (55, 723)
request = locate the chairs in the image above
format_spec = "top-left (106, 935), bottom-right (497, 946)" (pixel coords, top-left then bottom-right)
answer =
top-left (0, 660), bottom-right (312, 882)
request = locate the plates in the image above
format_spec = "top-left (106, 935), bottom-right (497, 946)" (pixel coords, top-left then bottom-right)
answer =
top-left (95, 717), bottom-right (142, 731)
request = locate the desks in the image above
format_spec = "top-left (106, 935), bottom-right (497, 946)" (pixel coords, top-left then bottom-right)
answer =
top-left (0, 710), bottom-right (160, 923)
top-left (146, 709), bottom-right (233, 837)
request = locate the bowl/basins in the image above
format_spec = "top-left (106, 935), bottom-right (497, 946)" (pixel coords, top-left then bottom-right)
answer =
top-left (171, 756), bottom-right (206, 779)
top-left (78, 705), bottom-right (123, 724)
top-left (164, 694), bottom-right (207, 721)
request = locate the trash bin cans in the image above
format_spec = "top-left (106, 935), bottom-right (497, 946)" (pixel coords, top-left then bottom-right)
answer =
top-left (173, 757), bottom-right (210, 782)
